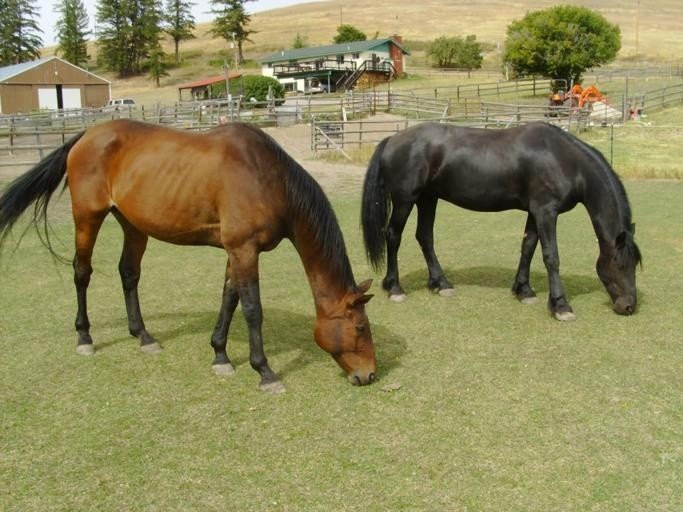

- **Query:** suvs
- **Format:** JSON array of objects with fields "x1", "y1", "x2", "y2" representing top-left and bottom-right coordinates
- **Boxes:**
[{"x1": 100, "y1": 97, "x2": 137, "y2": 110}]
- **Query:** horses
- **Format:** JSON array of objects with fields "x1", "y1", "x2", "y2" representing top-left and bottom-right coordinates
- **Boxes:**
[
  {"x1": 360, "y1": 120, "x2": 644, "y2": 322},
  {"x1": 0, "y1": 119, "x2": 377, "y2": 395}
]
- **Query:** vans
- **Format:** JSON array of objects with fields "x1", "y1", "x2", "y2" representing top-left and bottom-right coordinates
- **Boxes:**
[{"x1": 309, "y1": 78, "x2": 335, "y2": 93}]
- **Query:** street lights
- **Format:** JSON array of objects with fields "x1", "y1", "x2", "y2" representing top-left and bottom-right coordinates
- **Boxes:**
[{"x1": 229, "y1": 32, "x2": 238, "y2": 73}]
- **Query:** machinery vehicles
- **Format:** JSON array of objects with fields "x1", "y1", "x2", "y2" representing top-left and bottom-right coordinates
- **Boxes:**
[{"x1": 546, "y1": 78, "x2": 608, "y2": 116}]
